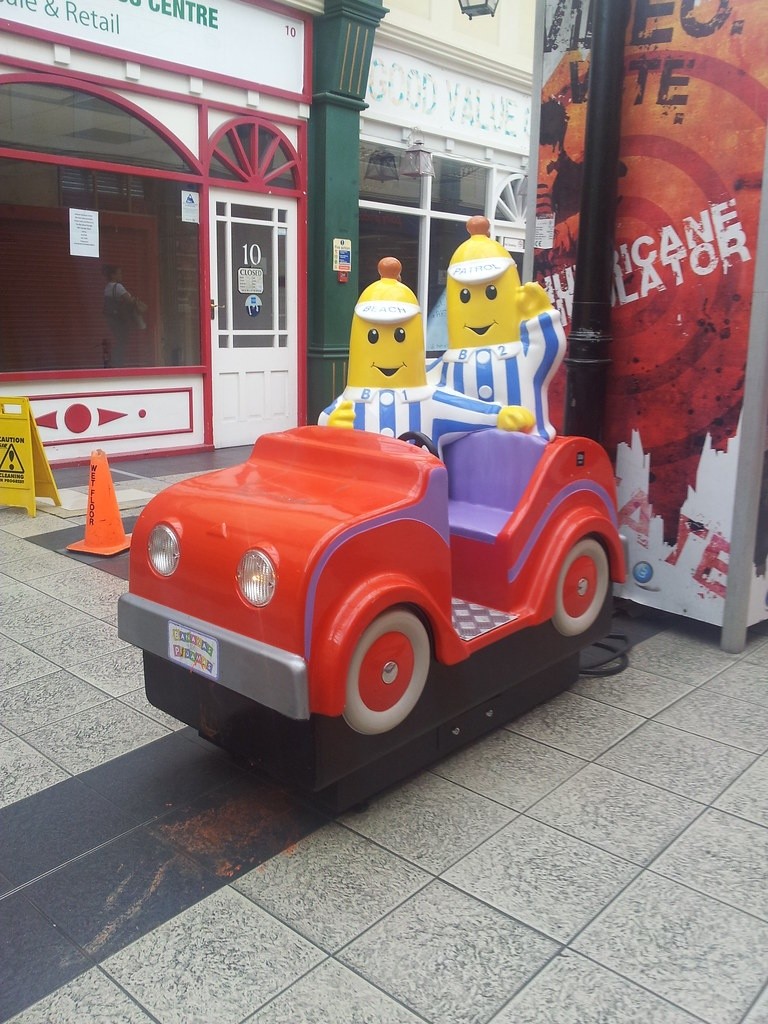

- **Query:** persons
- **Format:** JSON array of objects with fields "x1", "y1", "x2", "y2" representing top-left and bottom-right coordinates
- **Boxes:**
[
  {"x1": 318, "y1": 257, "x2": 538, "y2": 465},
  {"x1": 426, "y1": 215, "x2": 567, "y2": 443},
  {"x1": 101, "y1": 265, "x2": 140, "y2": 367}
]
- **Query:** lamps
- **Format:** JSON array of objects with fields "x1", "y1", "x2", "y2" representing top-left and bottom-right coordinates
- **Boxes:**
[
  {"x1": 365, "y1": 147, "x2": 399, "y2": 182},
  {"x1": 400, "y1": 127, "x2": 435, "y2": 177}
]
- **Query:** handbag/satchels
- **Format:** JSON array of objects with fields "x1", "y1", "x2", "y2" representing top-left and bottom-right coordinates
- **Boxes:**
[{"x1": 102, "y1": 282, "x2": 123, "y2": 320}]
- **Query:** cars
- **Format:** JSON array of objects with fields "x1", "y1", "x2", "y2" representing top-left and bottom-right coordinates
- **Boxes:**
[{"x1": 125, "y1": 389, "x2": 631, "y2": 739}]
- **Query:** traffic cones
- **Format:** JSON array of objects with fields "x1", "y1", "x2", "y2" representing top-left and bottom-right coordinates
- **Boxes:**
[{"x1": 66, "y1": 450, "x2": 133, "y2": 556}]
